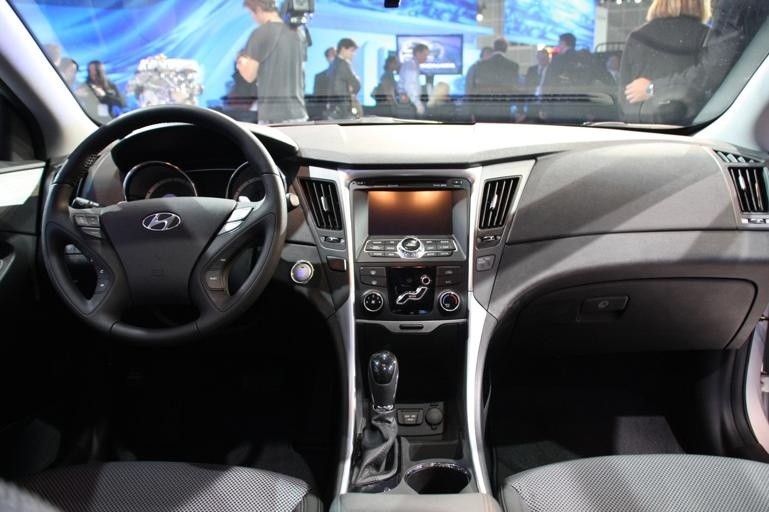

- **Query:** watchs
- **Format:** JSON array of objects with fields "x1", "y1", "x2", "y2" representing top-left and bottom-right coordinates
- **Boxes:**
[{"x1": 646, "y1": 84, "x2": 655, "y2": 97}]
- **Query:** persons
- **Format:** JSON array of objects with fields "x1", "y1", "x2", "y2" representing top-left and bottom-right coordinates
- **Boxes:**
[
  {"x1": 313, "y1": 47, "x2": 336, "y2": 94},
  {"x1": 376, "y1": 44, "x2": 457, "y2": 122},
  {"x1": 327, "y1": 37, "x2": 363, "y2": 119},
  {"x1": 624, "y1": 1, "x2": 767, "y2": 124},
  {"x1": 623, "y1": 1, "x2": 711, "y2": 124},
  {"x1": 237, "y1": 1, "x2": 308, "y2": 127},
  {"x1": 465, "y1": 33, "x2": 621, "y2": 125},
  {"x1": 42, "y1": 45, "x2": 126, "y2": 124}
]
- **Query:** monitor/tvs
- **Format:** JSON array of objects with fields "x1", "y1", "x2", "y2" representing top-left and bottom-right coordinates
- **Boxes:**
[{"x1": 396, "y1": 34, "x2": 462, "y2": 84}]
